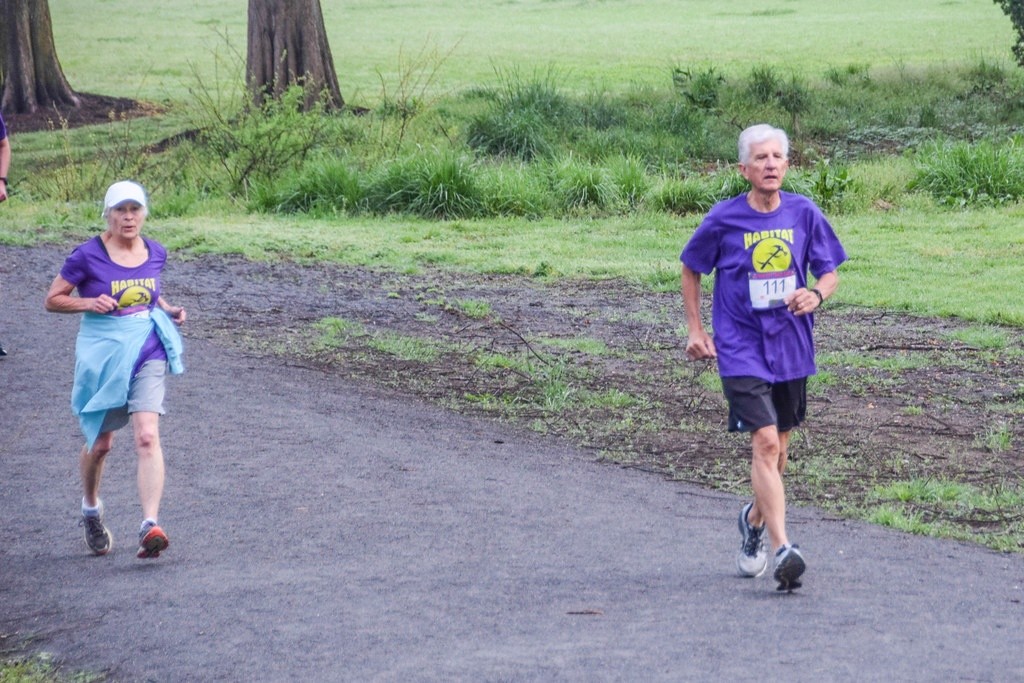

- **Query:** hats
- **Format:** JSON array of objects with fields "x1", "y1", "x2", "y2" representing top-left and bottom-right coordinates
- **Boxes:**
[{"x1": 105, "y1": 180, "x2": 144, "y2": 210}]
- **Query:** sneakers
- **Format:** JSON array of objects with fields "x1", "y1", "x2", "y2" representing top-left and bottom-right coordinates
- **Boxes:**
[
  {"x1": 81, "y1": 498, "x2": 111, "y2": 555},
  {"x1": 138, "y1": 524, "x2": 167, "y2": 559},
  {"x1": 738, "y1": 504, "x2": 769, "y2": 580},
  {"x1": 772, "y1": 542, "x2": 804, "y2": 591}
]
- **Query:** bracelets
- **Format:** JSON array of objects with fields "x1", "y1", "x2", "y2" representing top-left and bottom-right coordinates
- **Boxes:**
[
  {"x1": 0, "y1": 177, "x2": 8, "y2": 184},
  {"x1": 810, "y1": 289, "x2": 824, "y2": 303}
]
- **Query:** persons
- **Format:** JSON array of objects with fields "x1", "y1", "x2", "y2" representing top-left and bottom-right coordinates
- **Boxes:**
[
  {"x1": 46, "y1": 181, "x2": 188, "y2": 557},
  {"x1": 678, "y1": 122, "x2": 845, "y2": 591},
  {"x1": 0, "y1": 114, "x2": 12, "y2": 355}
]
